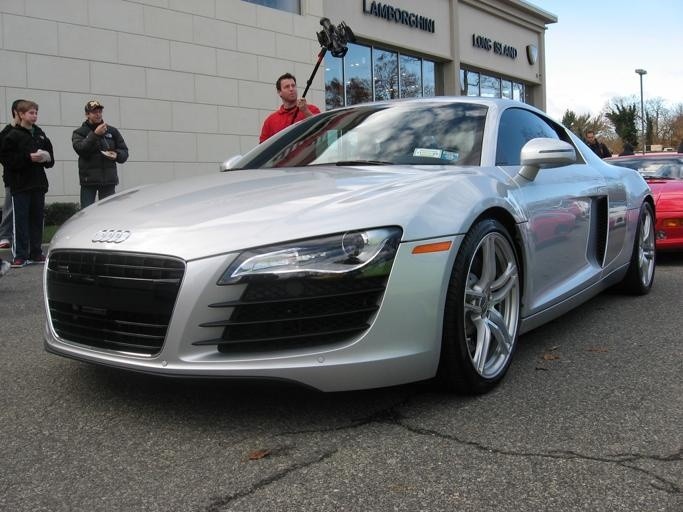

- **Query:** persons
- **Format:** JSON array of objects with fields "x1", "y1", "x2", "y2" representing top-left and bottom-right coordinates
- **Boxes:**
[
  {"x1": 584, "y1": 131, "x2": 612, "y2": 160},
  {"x1": 0, "y1": 99, "x2": 27, "y2": 249},
  {"x1": 72, "y1": 100, "x2": 128, "y2": 211},
  {"x1": 259, "y1": 73, "x2": 321, "y2": 167},
  {"x1": 0, "y1": 101, "x2": 54, "y2": 268}
]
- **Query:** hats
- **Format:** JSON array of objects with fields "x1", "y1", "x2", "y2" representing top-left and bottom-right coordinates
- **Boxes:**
[{"x1": 85, "y1": 100, "x2": 104, "y2": 112}]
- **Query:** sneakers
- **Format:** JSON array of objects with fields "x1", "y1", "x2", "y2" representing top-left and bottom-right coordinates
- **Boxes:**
[
  {"x1": 11, "y1": 258, "x2": 26, "y2": 267},
  {"x1": 0, "y1": 239, "x2": 10, "y2": 248},
  {"x1": 30, "y1": 256, "x2": 46, "y2": 264},
  {"x1": 0, "y1": 261, "x2": 10, "y2": 274}
]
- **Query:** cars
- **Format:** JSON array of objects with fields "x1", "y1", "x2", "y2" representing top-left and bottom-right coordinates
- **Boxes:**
[
  {"x1": 42, "y1": 95, "x2": 658, "y2": 397},
  {"x1": 602, "y1": 153, "x2": 683, "y2": 247}
]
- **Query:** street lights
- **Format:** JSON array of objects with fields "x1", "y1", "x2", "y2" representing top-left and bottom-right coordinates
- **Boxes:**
[{"x1": 636, "y1": 69, "x2": 646, "y2": 153}]
